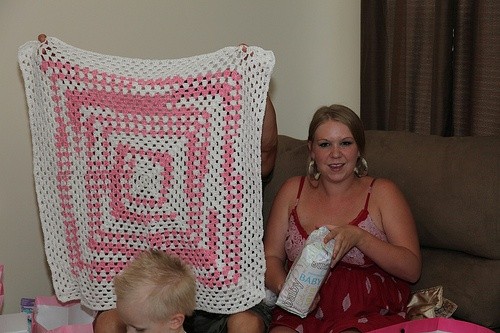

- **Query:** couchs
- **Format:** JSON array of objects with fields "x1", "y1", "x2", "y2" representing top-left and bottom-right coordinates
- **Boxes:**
[{"x1": 262, "y1": 128, "x2": 500, "y2": 328}]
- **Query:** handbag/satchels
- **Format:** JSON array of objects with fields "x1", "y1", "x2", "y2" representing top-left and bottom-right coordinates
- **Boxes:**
[{"x1": 31, "y1": 296, "x2": 100, "y2": 333}]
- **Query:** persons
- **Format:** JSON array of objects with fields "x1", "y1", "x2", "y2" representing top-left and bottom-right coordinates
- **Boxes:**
[
  {"x1": 264, "y1": 104, "x2": 421, "y2": 332},
  {"x1": 116, "y1": 246, "x2": 196, "y2": 333},
  {"x1": 36, "y1": 32, "x2": 278, "y2": 333}
]
